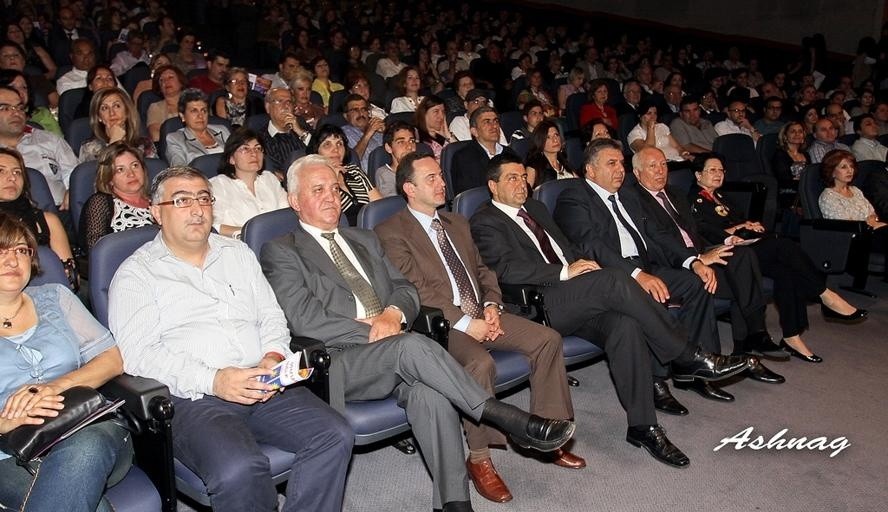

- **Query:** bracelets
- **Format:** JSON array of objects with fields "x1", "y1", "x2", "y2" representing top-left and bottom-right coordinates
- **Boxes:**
[
  {"x1": 299, "y1": 131, "x2": 308, "y2": 141},
  {"x1": 485, "y1": 303, "x2": 504, "y2": 315}
]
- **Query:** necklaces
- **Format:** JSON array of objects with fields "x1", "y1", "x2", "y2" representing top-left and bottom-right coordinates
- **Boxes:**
[{"x1": 0, "y1": 293, "x2": 25, "y2": 330}]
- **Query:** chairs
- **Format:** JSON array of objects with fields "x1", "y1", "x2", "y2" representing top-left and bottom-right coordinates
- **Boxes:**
[
  {"x1": 756, "y1": 133, "x2": 781, "y2": 175},
  {"x1": 109, "y1": 42, "x2": 126, "y2": 57},
  {"x1": 212, "y1": 89, "x2": 267, "y2": 116},
  {"x1": 67, "y1": 117, "x2": 92, "y2": 154},
  {"x1": 499, "y1": 111, "x2": 525, "y2": 142},
  {"x1": 242, "y1": 207, "x2": 450, "y2": 455},
  {"x1": 54, "y1": 64, "x2": 72, "y2": 77},
  {"x1": 162, "y1": 44, "x2": 180, "y2": 52},
  {"x1": 662, "y1": 113, "x2": 678, "y2": 124},
  {"x1": 91, "y1": 226, "x2": 333, "y2": 509},
  {"x1": 28, "y1": 168, "x2": 58, "y2": 211},
  {"x1": 664, "y1": 166, "x2": 774, "y2": 321},
  {"x1": 618, "y1": 114, "x2": 637, "y2": 138},
  {"x1": 28, "y1": 246, "x2": 176, "y2": 512},
  {"x1": 666, "y1": 167, "x2": 695, "y2": 217},
  {"x1": 440, "y1": 139, "x2": 471, "y2": 201},
  {"x1": 454, "y1": 186, "x2": 610, "y2": 387},
  {"x1": 310, "y1": 90, "x2": 323, "y2": 104},
  {"x1": 25, "y1": 66, "x2": 42, "y2": 74},
  {"x1": 533, "y1": 175, "x2": 584, "y2": 217},
  {"x1": 247, "y1": 113, "x2": 306, "y2": 133},
  {"x1": 368, "y1": 143, "x2": 433, "y2": 186},
  {"x1": 799, "y1": 162, "x2": 878, "y2": 302},
  {"x1": 127, "y1": 66, "x2": 148, "y2": 93},
  {"x1": 512, "y1": 139, "x2": 531, "y2": 162},
  {"x1": 711, "y1": 133, "x2": 780, "y2": 223},
  {"x1": 591, "y1": 77, "x2": 621, "y2": 97},
  {"x1": 346, "y1": 146, "x2": 362, "y2": 165},
  {"x1": 160, "y1": 115, "x2": 231, "y2": 160},
  {"x1": 386, "y1": 110, "x2": 418, "y2": 132},
  {"x1": 566, "y1": 92, "x2": 588, "y2": 127},
  {"x1": 358, "y1": 195, "x2": 545, "y2": 394},
  {"x1": 623, "y1": 172, "x2": 638, "y2": 188},
  {"x1": 137, "y1": 90, "x2": 157, "y2": 119},
  {"x1": 188, "y1": 152, "x2": 224, "y2": 179},
  {"x1": 563, "y1": 138, "x2": 584, "y2": 170},
  {"x1": 317, "y1": 114, "x2": 347, "y2": 128},
  {"x1": 57, "y1": 88, "x2": 90, "y2": 128},
  {"x1": 69, "y1": 158, "x2": 168, "y2": 233},
  {"x1": 857, "y1": 161, "x2": 887, "y2": 217},
  {"x1": 330, "y1": 90, "x2": 349, "y2": 114}
]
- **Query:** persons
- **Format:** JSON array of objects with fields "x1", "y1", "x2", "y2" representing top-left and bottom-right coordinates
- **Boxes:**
[
  {"x1": 108, "y1": 166, "x2": 355, "y2": 512},
  {"x1": 79, "y1": 144, "x2": 164, "y2": 257},
  {"x1": 631, "y1": 147, "x2": 791, "y2": 384},
  {"x1": 552, "y1": 137, "x2": 736, "y2": 416},
  {"x1": 317, "y1": 0, "x2": 374, "y2": 113},
  {"x1": 309, "y1": 123, "x2": 384, "y2": 225},
  {"x1": 374, "y1": 151, "x2": 586, "y2": 503},
  {"x1": 468, "y1": 154, "x2": 749, "y2": 468},
  {"x1": 0, "y1": 211, "x2": 134, "y2": 512},
  {"x1": 0, "y1": 147, "x2": 81, "y2": 294},
  {"x1": 342, "y1": 94, "x2": 386, "y2": 168},
  {"x1": 209, "y1": 126, "x2": 291, "y2": 242},
  {"x1": 817, "y1": 148, "x2": 888, "y2": 256},
  {"x1": 259, "y1": 153, "x2": 576, "y2": 512},
  {"x1": 701, "y1": 1, "x2": 888, "y2": 176},
  {"x1": 687, "y1": 151, "x2": 871, "y2": 364}
]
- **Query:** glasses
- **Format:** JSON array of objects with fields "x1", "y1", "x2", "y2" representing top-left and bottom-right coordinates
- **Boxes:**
[
  {"x1": 0, "y1": 103, "x2": 28, "y2": 115},
  {"x1": 235, "y1": 145, "x2": 265, "y2": 154},
  {"x1": 94, "y1": 74, "x2": 114, "y2": 82},
  {"x1": 1, "y1": 248, "x2": 35, "y2": 261},
  {"x1": 471, "y1": 99, "x2": 488, "y2": 105},
  {"x1": 270, "y1": 98, "x2": 293, "y2": 105},
  {"x1": 159, "y1": 195, "x2": 217, "y2": 206},
  {"x1": 729, "y1": 108, "x2": 746, "y2": 113},
  {"x1": 225, "y1": 77, "x2": 250, "y2": 87},
  {"x1": 702, "y1": 167, "x2": 727, "y2": 173}
]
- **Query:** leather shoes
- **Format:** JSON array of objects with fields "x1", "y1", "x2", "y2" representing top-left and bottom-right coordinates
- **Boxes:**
[
  {"x1": 682, "y1": 375, "x2": 736, "y2": 403},
  {"x1": 465, "y1": 455, "x2": 514, "y2": 504},
  {"x1": 548, "y1": 447, "x2": 587, "y2": 469},
  {"x1": 513, "y1": 414, "x2": 576, "y2": 456},
  {"x1": 653, "y1": 381, "x2": 689, "y2": 417},
  {"x1": 819, "y1": 305, "x2": 873, "y2": 325},
  {"x1": 673, "y1": 345, "x2": 750, "y2": 386},
  {"x1": 780, "y1": 337, "x2": 825, "y2": 364},
  {"x1": 747, "y1": 334, "x2": 789, "y2": 360},
  {"x1": 735, "y1": 356, "x2": 786, "y2": 385},
  {"x1": 626, "y1": 425, "x2": 689, "y2": 468}
]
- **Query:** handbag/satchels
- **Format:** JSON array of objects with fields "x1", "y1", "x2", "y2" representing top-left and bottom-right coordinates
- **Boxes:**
[{"x1": 3, "y1": 385, "x2": 106, "y2": 475}]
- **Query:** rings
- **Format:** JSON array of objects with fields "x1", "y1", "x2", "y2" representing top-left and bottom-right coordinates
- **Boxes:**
[{"x1": 27, "y1": 387, "x2": 38, "y2": 395}]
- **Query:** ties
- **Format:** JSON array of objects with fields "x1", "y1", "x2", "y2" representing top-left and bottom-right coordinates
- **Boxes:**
[
  {"x1": 321, "y1": 231, "x2": 382, "y2": 319},
  {"x1": 608, "y1": 194, "x2": 653, "y2": 273},
  {"x1": 431, "y1": 219, "x2": 483, "y2": 318},
  {"x1": 517, "y1": 207, "x2": 564, "y2": 266},
  {"x1": 656, "y1": 191, "x2": 704, "y2": 253}
]
